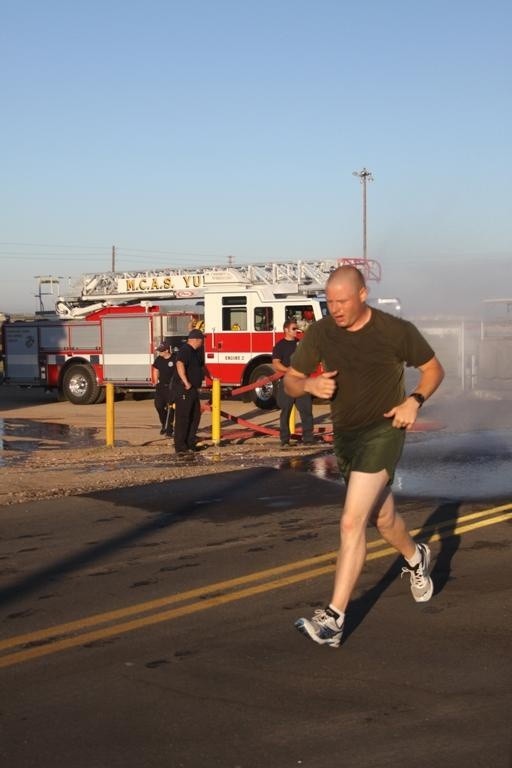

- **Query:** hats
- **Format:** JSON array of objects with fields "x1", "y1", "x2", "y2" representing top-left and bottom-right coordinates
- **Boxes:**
[
  {"x1": 188, "y1": 329, "x2": 206, "y2": 338},
  {"x1": 155, "y1": 342, "x2": 170, "y2": 351}
]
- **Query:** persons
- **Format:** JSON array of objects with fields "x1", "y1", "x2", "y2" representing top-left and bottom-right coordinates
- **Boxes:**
[
  {"x1": 271, "y1": 319, "x2": 319, "y2": 448},
  {"x1": 173, "y1": 328, "x2": 213, "y2": 460},
  {"x1": 281, "y1": 267, "x2": 447, "y2": 651},
  {"x1": 152, "y1": 342, "x2": 176, "y2": 437}
]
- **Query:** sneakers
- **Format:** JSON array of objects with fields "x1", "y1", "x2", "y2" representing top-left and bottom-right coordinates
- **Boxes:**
[
  {"x1": 295, "y1": 605, "x2": 345, "y2": 648},
  {"x1": 400, "y1": 543, "x2": 434, "y2": 602}
]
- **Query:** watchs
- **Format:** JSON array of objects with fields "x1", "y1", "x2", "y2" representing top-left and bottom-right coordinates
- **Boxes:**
[{"x1": 408, "y1": 392, "x2": 425, "y2": 409}]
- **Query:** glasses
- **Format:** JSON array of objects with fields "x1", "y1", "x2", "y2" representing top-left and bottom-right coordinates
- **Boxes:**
[{"x1": 292, "y1": 328, "x2": 297, "y2": 330}]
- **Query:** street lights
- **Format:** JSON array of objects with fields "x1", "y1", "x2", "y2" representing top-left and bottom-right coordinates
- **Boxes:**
[{"x1": 353, "y1": 167, "x2": 375, "y2": 258}]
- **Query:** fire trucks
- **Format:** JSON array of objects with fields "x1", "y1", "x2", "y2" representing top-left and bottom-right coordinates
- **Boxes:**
[{"x1": 0, "y1": 258, "x2": 402, "y2": 409}]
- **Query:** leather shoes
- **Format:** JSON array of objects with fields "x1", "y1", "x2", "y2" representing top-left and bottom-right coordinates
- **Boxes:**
[
  {"x1": 192, "y1": 443, "x2": 207, "y2": 452},
  {"x1": 159, "y1": 426, "x2": 166, "y2": 435}
]
